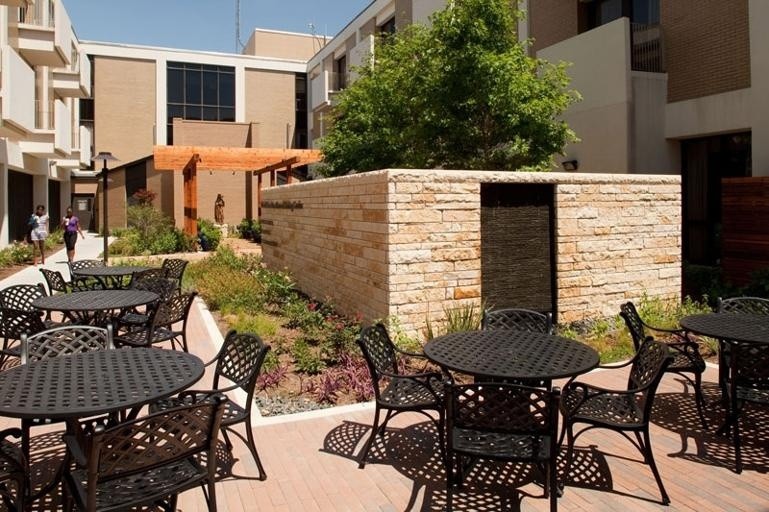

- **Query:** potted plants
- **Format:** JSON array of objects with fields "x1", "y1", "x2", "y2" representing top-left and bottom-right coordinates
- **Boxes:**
[{"x1": 197, "y1": 218, "x2": 223, "y2": 251}]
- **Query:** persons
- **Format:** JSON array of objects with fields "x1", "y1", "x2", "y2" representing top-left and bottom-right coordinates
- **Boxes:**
[
  {"x1": 27, "y1": 205, "x2": 49, "y2": 268},
  {"x1": 61, "y1": 207, "x2": 85, "y2": 264}
]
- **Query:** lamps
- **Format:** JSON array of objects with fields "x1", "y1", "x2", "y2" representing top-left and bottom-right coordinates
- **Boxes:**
[{"x1": 562, "y1": 160, "x2": 578, "y2": 171}]
